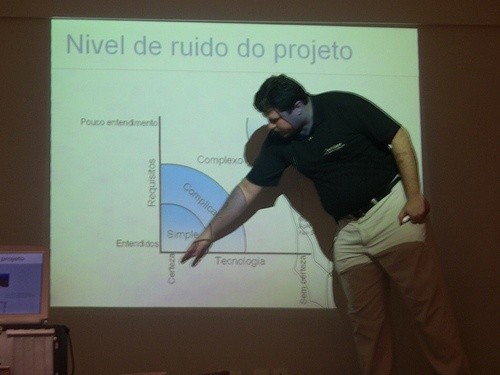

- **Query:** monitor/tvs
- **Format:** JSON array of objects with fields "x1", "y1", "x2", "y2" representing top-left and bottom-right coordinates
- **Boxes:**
[{"x1": 0, "y1": 244, "x2": 50, "y2": 327}]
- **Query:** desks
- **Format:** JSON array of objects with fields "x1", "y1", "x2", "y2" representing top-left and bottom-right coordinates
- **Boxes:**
[{"x1": 0, "y1": 328, "x2": 68, "y2": 375}]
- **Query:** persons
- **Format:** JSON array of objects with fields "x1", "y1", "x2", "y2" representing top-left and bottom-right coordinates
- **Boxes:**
[{"x1": 180, "y1": 74, "x2": 473, "y2": 375}]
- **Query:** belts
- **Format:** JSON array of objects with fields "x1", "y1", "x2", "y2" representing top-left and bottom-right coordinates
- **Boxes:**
[{"x1": 336, "y1": 176, "x2": 402, "y2": 224}]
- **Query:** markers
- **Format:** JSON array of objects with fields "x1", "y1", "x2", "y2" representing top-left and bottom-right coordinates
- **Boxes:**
[{"x1": 400, "y1": 215, "x2": 411, "y2": 224}]
